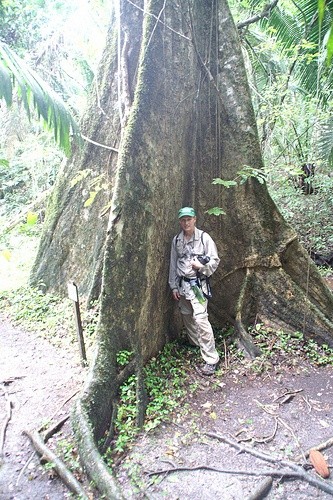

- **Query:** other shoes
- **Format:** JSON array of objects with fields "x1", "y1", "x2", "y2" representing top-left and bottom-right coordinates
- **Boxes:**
[{"x1": 202, "y1": 364, "x2": 217, "y2": 375}]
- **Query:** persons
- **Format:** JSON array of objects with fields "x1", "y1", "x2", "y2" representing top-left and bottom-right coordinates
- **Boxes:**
[{"x1": 167, "y1": 206, "x2": 220, "y2": 378}]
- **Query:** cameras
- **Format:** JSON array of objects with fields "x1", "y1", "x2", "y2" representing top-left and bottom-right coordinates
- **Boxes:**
[{"x1": 197, "y1": 255, "x2": 210, "y2": 265}]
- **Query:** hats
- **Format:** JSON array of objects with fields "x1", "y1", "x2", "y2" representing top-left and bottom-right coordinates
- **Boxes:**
[{"x1": 178, "y1": 207, "x2": 196, "y2": 219}]
